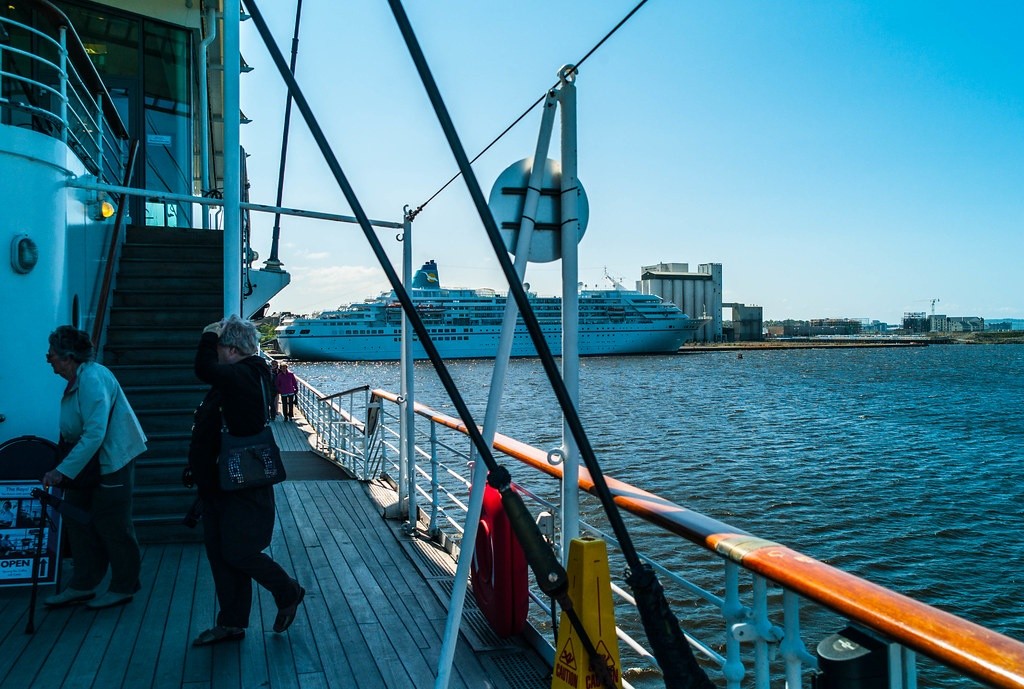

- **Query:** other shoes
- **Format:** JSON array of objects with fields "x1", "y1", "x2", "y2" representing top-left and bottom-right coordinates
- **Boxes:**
[
  {"x1": 86, "y1": 591, "x2": 133, "y2": 610},
  {"x1": 285, "y1": 417, "x2": 288, "y2": 421},
  {"x1": 42, "y1": 586, "x2": 97, "y2": 606},
  {"x1": 272, "y1": 417, "x2": 275, "y2": 420},
  {"x1": 276, "y1": 413, "x2": 279, "y2": 415}
]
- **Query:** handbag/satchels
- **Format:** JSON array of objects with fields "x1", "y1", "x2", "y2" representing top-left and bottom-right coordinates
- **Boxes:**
[
  {"x1": 218, "y1": 373, "x2": 287, "y2": 493},
  {"x1": 57, "y1": 440, "x2": 101, "y2": 487}
]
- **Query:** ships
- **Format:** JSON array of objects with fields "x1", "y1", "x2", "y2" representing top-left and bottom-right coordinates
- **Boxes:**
[{"x1": 274, "y1": 260, "x2": 713, "y2": 361}]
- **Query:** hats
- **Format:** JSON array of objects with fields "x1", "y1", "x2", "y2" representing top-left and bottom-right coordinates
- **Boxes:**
[{"x1": 49, "y1": 325, "x2": 93, "y2": 355}]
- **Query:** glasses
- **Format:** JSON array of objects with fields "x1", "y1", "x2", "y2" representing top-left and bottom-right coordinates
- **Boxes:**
[{"x1": 46, "y1": 353, "x2": 58, "y2": 360}]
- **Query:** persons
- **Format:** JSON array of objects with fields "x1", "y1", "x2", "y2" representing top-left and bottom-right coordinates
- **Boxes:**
[
  {"x1": 41, "y1": 326, "x2": 147, "y2": 609},
  {"x1": 187, "y1": 316, "x2": 306, "y2": 648},
  {"x1": 265, "y1": 360, "x2": 299, "y2": 422}
]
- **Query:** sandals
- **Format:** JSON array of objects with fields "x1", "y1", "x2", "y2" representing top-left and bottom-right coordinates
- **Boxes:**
[
  {"x1": 273, "y1": 587, "x2": 305, "y2": 633},
  {"x1": 193, "y1": 626, "x2": 245, "y2": 647}
]
